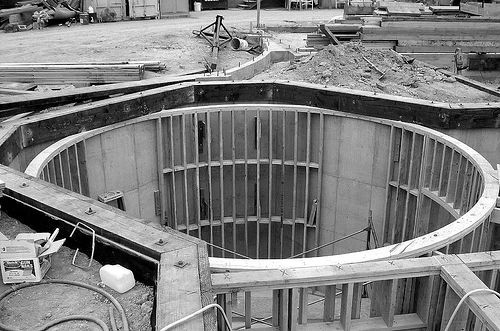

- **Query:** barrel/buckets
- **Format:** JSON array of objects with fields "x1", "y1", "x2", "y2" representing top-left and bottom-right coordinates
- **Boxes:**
[
  {"x1": 230, "y1": 38, "x2": 248, "y2": 51},
  {"x1": 194, "y1": 2, "x2": 202, "y2": 12},
  {"x1": 80, "y1": 14, "x2": 89, "y2": 24},
  {"x1": 88, "y1": 12, "x2": 97, "y2": 24}
]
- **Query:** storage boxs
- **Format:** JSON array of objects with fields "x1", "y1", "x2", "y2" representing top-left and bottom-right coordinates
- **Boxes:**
[{"x1": 1, "y1": 228, "x2": 66, "y2": 286}]
